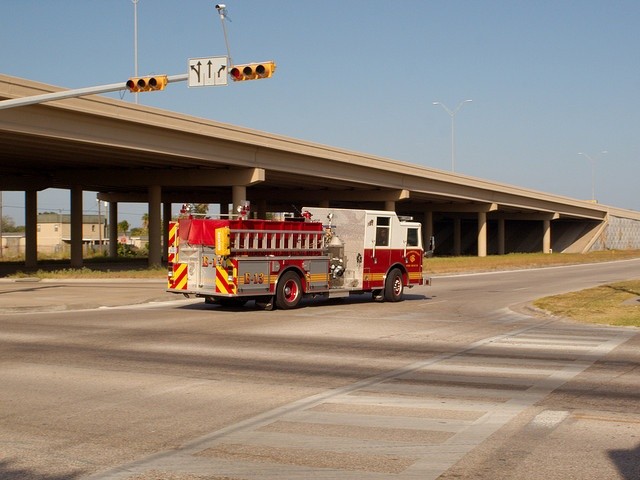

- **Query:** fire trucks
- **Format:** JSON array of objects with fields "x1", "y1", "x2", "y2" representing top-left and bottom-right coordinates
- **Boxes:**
[{"x1": 167, "y1": 201, "x2": 435, "y2": 311}]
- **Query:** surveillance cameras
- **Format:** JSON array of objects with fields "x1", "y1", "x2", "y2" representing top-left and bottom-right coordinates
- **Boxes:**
[{"x1": 215, "y1": 4, "x2": 226, "y2": 10}]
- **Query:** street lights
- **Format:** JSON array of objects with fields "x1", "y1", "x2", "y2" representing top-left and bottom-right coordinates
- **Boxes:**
[
  {"x1": 433, "y1": 99, "x2": 472, "y2": 171},
  {"x1": 578, "y1": 151, "x2": 606, "y2": 199}
]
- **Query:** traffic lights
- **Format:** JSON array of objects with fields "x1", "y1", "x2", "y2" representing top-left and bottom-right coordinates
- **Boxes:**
[
  {"x1": 126, "y1": 74, "x2": 168, "y2": 92},
  {"x1": 230, "y1": 61, "x2": 276, "y2": 81}
]
